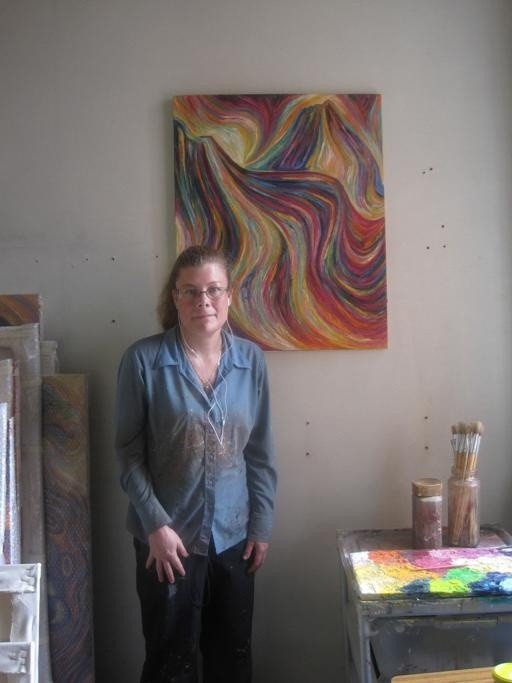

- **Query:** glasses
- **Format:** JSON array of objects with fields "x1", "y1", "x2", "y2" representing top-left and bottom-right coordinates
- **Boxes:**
[{"x1": 173, "y1": 286, "x2": 229, "y2": 300}]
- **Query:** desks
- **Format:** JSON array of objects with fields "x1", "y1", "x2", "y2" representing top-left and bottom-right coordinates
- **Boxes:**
[{"x1": 336, "y1": 523, "x2": 512, "y2": 683}]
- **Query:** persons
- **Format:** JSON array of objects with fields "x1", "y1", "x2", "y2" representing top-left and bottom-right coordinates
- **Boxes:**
[{"x1": 111, "y1": 244, "x2": 280, "y2": 683}]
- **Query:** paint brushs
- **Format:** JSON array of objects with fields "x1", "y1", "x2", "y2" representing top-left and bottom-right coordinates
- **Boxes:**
[{"x1": 449, "y1": 421, "x2": 485, "y2": 547}]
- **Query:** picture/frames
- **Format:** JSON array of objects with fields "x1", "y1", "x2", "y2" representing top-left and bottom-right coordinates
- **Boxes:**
[{"x1": 172, "y1": 93, "x2": 390, "y2": 354}]
